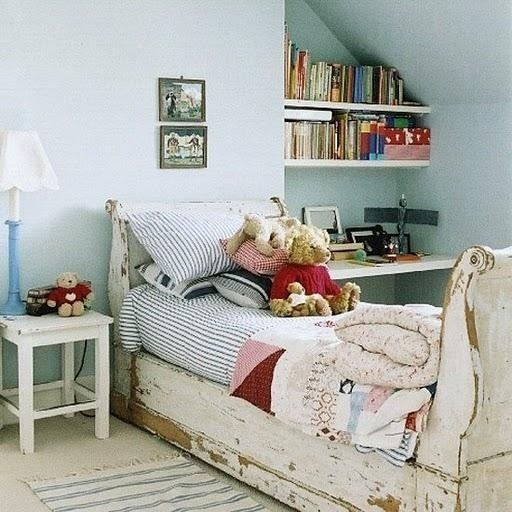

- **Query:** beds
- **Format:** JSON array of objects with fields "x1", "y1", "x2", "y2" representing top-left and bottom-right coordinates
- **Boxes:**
[{"x1": 105, "y1": 190, "x2": 510, "y2": 511}]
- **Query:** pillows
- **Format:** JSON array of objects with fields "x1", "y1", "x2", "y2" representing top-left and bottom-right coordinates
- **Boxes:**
[
  {"x1": 132, "y1": 258, "x2": 222, "y2": 302},
  {"x1": 216, "y1": 233, "x2": 292, "y2": 280},
  {"x1": 207, "y1": 266, "x2": 279, "y2": 309},
  {"x1": 117, "y1": 201, "x2": 256, "y2": 289}
]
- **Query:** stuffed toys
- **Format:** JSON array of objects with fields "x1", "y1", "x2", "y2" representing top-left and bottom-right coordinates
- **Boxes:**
[
  {"x1": 269, "y1": 228, "x2": 362, "y2": 318},
  {"x1": 287, "y1": 281, "x2": 309, "y2": 317},
  {"x1": 47, "y1": 272, "x2": 94, "y2": 317},
  {"x1": 225, "y1": 211, "x2": 301, "y2": 257}
]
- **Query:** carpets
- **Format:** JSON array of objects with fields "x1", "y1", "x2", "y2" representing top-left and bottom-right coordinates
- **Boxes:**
[{"x1": 16, "y1": 448, "x2": 274, "y2": 511}]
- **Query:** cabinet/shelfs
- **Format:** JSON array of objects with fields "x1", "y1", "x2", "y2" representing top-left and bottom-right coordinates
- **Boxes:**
[{"x1": 282, "y1": 89, "x2": 434, "y2": 169}]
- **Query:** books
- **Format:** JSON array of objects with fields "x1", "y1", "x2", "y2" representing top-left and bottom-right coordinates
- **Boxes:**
[
  {"x1": 327, "y1": 242, "x2": 365, "y2": 250},
  {"x1": 331, "y1": 251, "x2": 357, "y2": 261},
  {"x1": 284, "y1": 20, "x2": 417, "y2": 159}
]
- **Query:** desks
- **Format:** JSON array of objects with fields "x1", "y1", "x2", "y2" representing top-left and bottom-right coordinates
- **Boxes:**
[
  {"x1": 321, "y1": 247, "x2": 463, "y2": 283},
  {"x1": 0, "y1": 307, "x2": 119, "y2": 457}
]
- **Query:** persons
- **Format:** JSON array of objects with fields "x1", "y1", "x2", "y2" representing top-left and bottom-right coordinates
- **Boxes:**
[{"x1": 384, "y1": 241, "x2": 399, "y2": 264}]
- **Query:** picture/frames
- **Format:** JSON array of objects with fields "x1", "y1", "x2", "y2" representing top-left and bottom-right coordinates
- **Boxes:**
[
  {"x1": 158, "y1": 75, "x2": 207, "y2": 124},
  {"x1": 301, "y1": 203, "x2": 413, "y2": 257},
  {"x1": 159, "y1": 124, "x2": 209, "y2": 170}
]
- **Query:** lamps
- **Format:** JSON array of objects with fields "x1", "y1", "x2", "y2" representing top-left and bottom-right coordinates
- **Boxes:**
[{"x1": 1, "y1": 124, "x2": 66, "y2": 317}]
